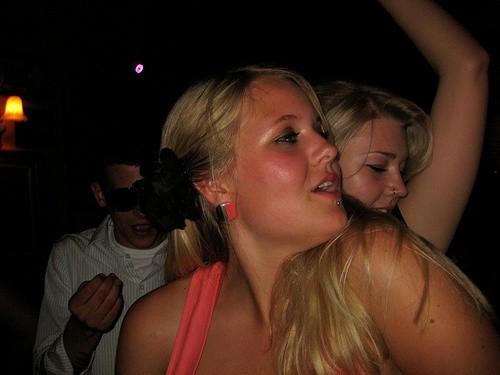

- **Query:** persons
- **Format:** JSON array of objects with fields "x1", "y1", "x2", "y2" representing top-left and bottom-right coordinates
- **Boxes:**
[
  {"x1": 114, "y1": 69, "x2": 500, "y2": 375},
  {"x1": 312, "y1": 0, "x2": 489, "y2": 255},
  {"x1": 33, "y1": 144, "x2": 170, "y2": 375}
]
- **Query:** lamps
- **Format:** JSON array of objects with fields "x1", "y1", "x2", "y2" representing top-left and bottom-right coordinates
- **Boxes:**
[{"x1": 1, "y1": 80, "x2": 28, "y2": 122}]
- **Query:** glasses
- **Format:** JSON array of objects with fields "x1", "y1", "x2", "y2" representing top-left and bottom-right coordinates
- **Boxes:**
[{"x1": 98, "y1": 182, "x2": 139, "y2": 213}]
